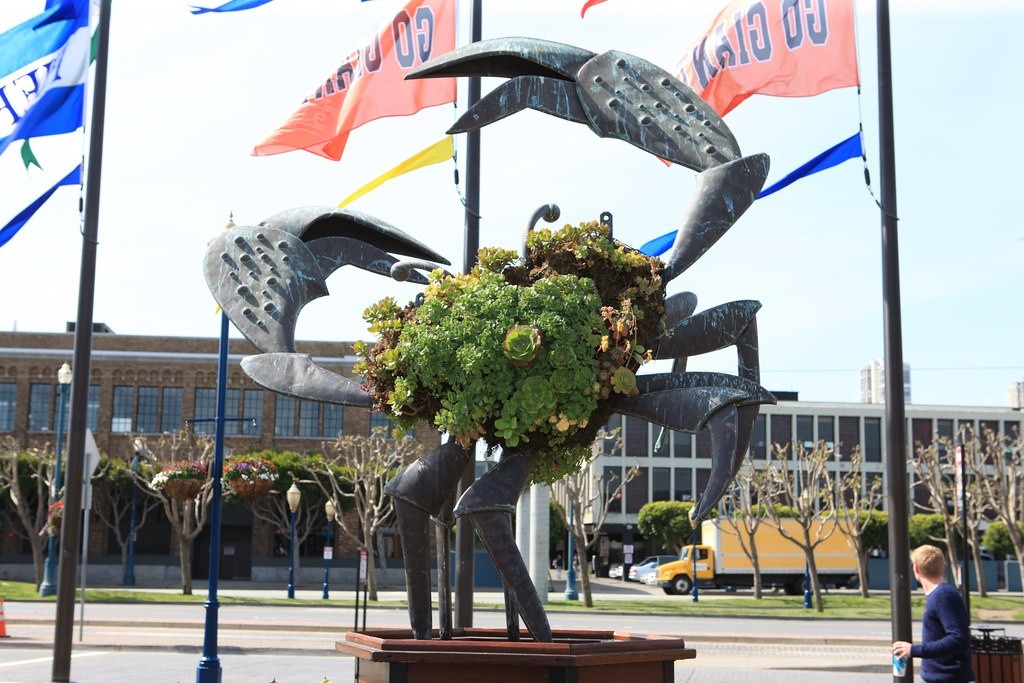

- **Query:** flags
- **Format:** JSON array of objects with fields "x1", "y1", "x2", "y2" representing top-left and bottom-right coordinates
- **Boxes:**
[
  {"x1": 0, "y1": 0, "x2": 92, "y2": 152},
  {"x1": 658, "y1": 0, "x2": 860, "y2": 167},
  {"x1": 251, "y1": 0, "x2": 457, "y2": 161}
]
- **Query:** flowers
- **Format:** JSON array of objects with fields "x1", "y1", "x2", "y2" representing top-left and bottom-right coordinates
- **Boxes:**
[
  {"x1": 223, "y1": 457, "x2": 280, "y2": 485},
  {"x1": 49, "y1": 501, "x2": 63, "y2": 519},
  {"x1": 148, "y1": 461, "x2": 206, "y2": 491}
]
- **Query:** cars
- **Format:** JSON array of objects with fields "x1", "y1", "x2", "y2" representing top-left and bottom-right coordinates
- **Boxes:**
[{"x1": 627, "y1": 556, "x2": 657, "y2": 585}]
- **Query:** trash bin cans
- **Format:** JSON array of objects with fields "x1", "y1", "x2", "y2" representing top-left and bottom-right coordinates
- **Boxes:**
[{"x1": 969, "y1": 626, "x2": 1024, "y2": 683}]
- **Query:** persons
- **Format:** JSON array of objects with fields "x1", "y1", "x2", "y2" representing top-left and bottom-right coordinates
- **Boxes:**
[
  {"x1": 892, "y1": 545, "x2": 976, "y2": 683},
  {"x1": 556, "y1": 555, "x2": 562, "y2": 578},
  {"x1": 697, "y1": 550, "x2": 707, "y2": 559}
]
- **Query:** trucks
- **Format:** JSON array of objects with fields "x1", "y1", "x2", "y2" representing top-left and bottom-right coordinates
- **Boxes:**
[{"x1": 654, "y1": 516, "x2": 860, "y2": 596}]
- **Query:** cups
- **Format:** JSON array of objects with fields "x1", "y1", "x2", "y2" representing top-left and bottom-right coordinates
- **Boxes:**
[{"x1": 892, "y1": 651, "x2": 907, "y2": 677}]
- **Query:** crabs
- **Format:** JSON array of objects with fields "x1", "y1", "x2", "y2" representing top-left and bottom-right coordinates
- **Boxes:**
[{"x1": 201, "y1": 35, "x2": 778, "y2": 643}]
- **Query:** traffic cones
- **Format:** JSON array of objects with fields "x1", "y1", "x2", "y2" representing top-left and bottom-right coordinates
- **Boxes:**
[{"x1": 0, "y1": 598, "x2": 11, "y2": 639}]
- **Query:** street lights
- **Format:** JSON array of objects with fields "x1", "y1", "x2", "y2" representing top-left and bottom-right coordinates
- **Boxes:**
[
  {"x1": 687, "y1": 504, "x2": 699, "y2": 603},
  {"x1": 286, "y1": 482, "x2": 302, "y2": 597},
  {"x1": 39, "y1": 363, "x2": 74, "y2": 598},
  {"x1": 122, "y1": 436, "x2": 143, "y2": 586},
  {"x1": 322, "y1": 496, "x2": 337, "y2": 598}
]
